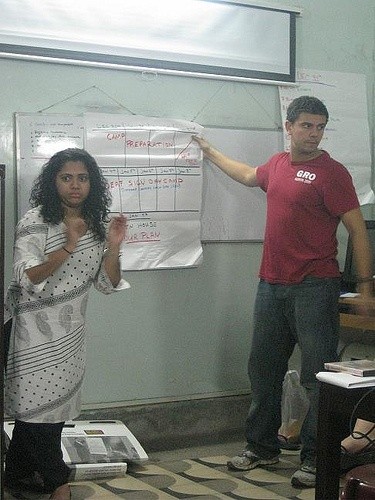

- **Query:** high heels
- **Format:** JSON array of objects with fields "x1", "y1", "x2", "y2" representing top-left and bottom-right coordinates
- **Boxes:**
[{"x1": 339, "y1": 431, "x2": 375, "y2": 468}]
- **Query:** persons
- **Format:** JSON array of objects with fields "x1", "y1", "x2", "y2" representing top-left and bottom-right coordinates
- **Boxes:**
[
  {"x1": 73, "y1": 437, "x2": 90, "y2": 463},
  {"x1": 131, "y1": 447, "x2": 140, "y2": 463},
  {"x1": 190, "y1": 95, "x2": 375, "y2": 489},
  {"x1": 5, "y1": 148, "x2": 131, "y2": 500}
]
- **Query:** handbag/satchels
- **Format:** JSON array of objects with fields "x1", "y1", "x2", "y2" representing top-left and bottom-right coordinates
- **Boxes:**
[{"x1": 279, "y1": 370, "x2": 309, "y2": 442}]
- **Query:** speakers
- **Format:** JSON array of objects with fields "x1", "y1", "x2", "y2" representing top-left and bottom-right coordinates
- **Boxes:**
[{"x1": 343, "y1": 220, "x2": 375, "y2": 293}]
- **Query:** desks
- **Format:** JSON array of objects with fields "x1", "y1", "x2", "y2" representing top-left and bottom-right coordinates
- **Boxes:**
[
  {"x1": 338, "y1": 295, "x2": 375, "y2": 331},
  {"x1": 314, "y1": 373, "x2": 375, "y2": 500}
]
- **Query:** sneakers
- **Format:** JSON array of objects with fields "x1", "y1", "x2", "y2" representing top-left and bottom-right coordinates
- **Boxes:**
[
  {"x1": 227, "y1": 449, "x2": 279, "y2": 470},
  {"x1": 291, "y1": 464, "x2": 316, "y2": 487}
]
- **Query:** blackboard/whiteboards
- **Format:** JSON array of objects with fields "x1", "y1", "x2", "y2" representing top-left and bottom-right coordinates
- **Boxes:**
[
  {"x1": 16, "y1": 112, "x2": 160, "y2": 223},
  {"x1": 201, "y1": 125, "x2": 284, "y2": 243}
]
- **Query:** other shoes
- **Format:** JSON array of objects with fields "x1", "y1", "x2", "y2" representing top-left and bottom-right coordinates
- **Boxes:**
[
  {"x1": 50, "y1": 484, "x2": 71, "y2": 500},
  {"x1": 11, "y1": 472, "x2": 45, "y2": 490}
]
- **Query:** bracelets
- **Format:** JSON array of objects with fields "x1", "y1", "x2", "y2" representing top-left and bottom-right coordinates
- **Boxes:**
[
  {"x1": 358, "y1": 275, "x2": 372, "y2": 283},
  {"x1": 62, "y1": 246, "x2": 73, "y2": 255}
]
